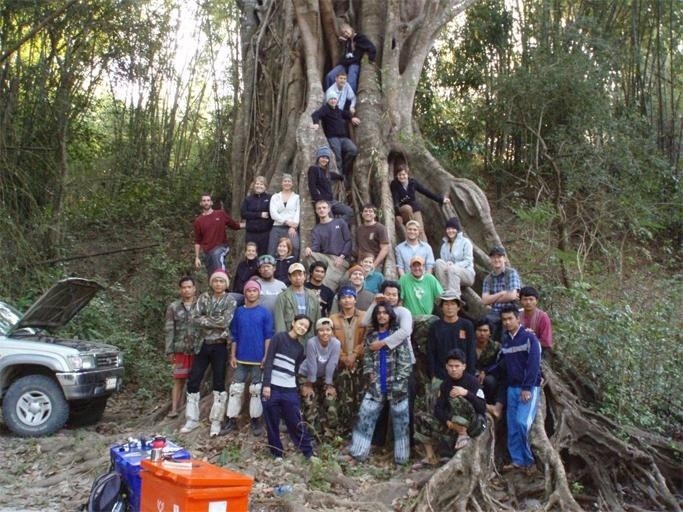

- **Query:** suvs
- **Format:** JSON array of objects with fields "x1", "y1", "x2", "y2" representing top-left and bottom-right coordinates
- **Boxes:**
[{"x1": 0, "y1": 272, "x2": 125, "y2": 436}]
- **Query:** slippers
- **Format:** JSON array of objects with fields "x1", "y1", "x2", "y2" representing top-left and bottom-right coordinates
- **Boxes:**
[
  {"x1": 527, "y1": 464, "x2": 537, "y2": 476},
  {"x1": 485, "y1": 402, "x2": 500, "y2": 419},
  {"x1": 503, "y1": 463, "x2": 521, "y2": 469},
  {"x1": 167, "y1": 410, "x2": 178, "y2": 418},
  {"x1": 454, "y1": 435, "x2": 471, "y2": 450},
  {"x1": 411, "y1": 459, "x2": 432, "y2": 469}
]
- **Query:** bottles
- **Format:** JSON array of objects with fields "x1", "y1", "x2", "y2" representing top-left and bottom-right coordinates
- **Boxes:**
[{"x1": 139, "y1": 433, "x2": 145, "y2": 447}]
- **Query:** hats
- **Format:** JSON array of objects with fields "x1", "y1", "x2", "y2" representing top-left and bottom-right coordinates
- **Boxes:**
[
  {"x1": 327, "y1": 92, "x2": 337, "y2": 101},
  {"x1": 317, "y1": 147, "x2": 331, "y2": 159},
  {"x1": 488, "y1": 246, "x2": 505, "y2": 256},
  {"x1": 257, "y1": 255, "x2": 275, "y2": 268},
  {"x1": 288, "y1": 263, "x2": 305, "y2": 273},
  {"x1": 348, "y1": 265, "x2": 364, "y2": 278},
  {"x1": 410, "y1": 256, "x2": 424, "y2": 266},
  {"x1": 243, "y1": 281, "x2": 260, "y2": 295},
  {"x1": 209, "y1": 269, "x2": 229, "y2": 289},
  {"x1": 446, "y1": 217, "x2": 460, "y2": 231},
  {"x1": 316, "y1": 317, "x2": 333, "y2": 330},
  {"x1": 337, "y1": 285, "x2": 357, "y2": 299},
  {"x1": 435, "y1": 290, "x2": 465, "y2": 305}
]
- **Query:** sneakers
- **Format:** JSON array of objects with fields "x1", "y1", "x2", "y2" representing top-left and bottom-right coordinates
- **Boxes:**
[
  {"x1": 208, "y1": 420, "x2": 220, "y2": 437},
  {"x1": 249, "y1": 419, "x2": 263, "y2": 437},
  {"x1": 220, "y1": 419, "x2": 240, "y2": 435},
  {"x1": 181, "y1": 419, "x2": 202, "y2": 432},
  {"x1": 304, "y1": 456, "x2": 323, "y2": 466},
  {"x1": 273, "y1": 455, "x2": 283, "y2": 469}
]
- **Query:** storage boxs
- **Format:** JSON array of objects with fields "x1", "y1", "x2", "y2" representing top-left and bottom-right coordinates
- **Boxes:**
[
  {"x1": 109, "y1": 437, "x2": 190, "y2": 511},
  {"x1": 136, "y1": 455, "x2": 254, "y2": 512}
]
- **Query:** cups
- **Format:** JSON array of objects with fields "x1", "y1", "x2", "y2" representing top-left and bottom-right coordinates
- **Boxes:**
[{"x1": 150, "y1": 449, "x2": 162, "y2": 460}]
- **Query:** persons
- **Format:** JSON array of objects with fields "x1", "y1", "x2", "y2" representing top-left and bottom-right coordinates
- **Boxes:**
[
  {"x1": 312, "y1": 91, "x2": 360, "y2": 177},
  {"x1": 326, "y1": 23, "x2": 376, "y2": 97},
  {"x1": 326, "y1": 72, "x2": 357, "y2": 116}
]
